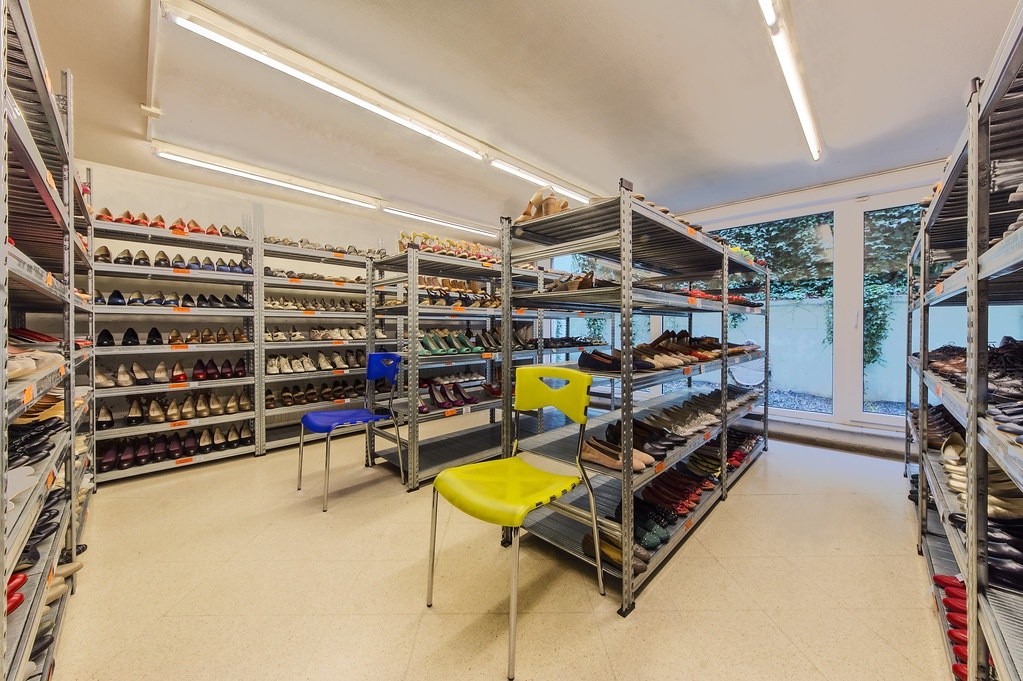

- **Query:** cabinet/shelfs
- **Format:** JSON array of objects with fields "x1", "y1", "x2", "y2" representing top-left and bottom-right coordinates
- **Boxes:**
[{"x1": 0, "y1": 0, "x2": 1023, "y2": 681}]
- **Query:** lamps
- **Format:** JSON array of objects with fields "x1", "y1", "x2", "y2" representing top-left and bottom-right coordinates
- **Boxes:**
[
  {"x1": 147, "y1": 139, "x2": 380, "y2": 212},
  {"x1": 758, "y1": 0, "x2": 791, "y2": 29},
  {"x1": 487, "y1": 145, "x2": 601, "y2": 203},
  {"x1": 766, "y1": 23, "x2": 822, "y2": 159},
  {"x1": 159, "y1": 1, "x2": 488, "y2": 161},
  {"x1": 379, "y1": 201, "x2": 502, "y2": 240}
]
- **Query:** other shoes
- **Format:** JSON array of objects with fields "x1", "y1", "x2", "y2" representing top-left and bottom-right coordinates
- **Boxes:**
[
  {"x1": 87, "y1": 358, "x2": 247, "y2": 388},
  {"x1": 99, "y1": 422, "x2": 254, "y2": 473},
  {"x1": 95, "y1": 206, "x2": 252, "y2": 240},
  {"x1": 95, "y1": 327, "x2": 250, "y2": 346},
  {"x1": 509, "y1": 184, "x2": 768, "y2": 372},
  {"x1": 913, "y1": 336, "x2": 1023, "y2": 447},
  {"x1": 910, "y1": 151, "x2": 1023, "y2": 300},
  {"x1": 940, "y1": 432, "x2": 1023, "y2": 595},
  {"x1": 932, "y1": 572, "x2": 999, "y2": 681},
  {"x1": 6, "y1": 385, "x2": 93, "y2": 513},
  {"x1": 94, "y1": 245, "x2": 254, "y2": 276},
  {"x1": 582, "y1": 383, "x2": 765, "y2": 573},
  {"x1": 5, "y1": 431, "x2": 95, "y2": 681},
  {"x1": 909, "y1": 403, "x2": 966, "y2": 450},
  {"x1": 265, "y1": 229, "x2": 612, "y2": 412},
  {"x1": 909, "y1": 472, "x2": 937, "y2": 511},
  {"x1": 95, "y1": 390, "x2": 253, "y2": 430},
  {"x1": 95, "y1": 287, "x2": 254, "y2": 310},
  {"x1": 7, "y1": 326, "x2": 65, "y2": 380}
]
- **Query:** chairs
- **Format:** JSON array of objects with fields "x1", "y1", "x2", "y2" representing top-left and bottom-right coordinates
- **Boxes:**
[
  {"x1": 426, "y1": 363, "x2": 606, "y2": 681},
  {"x1": 297, "y1": 353, "x2": 405, "y2": 512}
]
